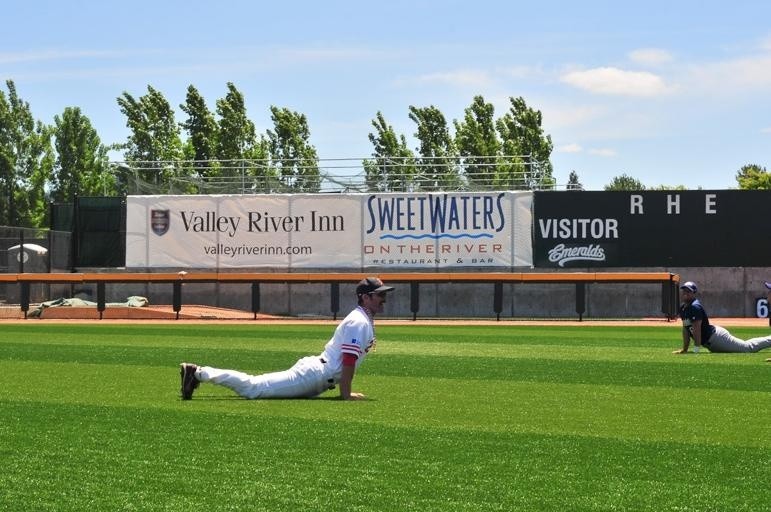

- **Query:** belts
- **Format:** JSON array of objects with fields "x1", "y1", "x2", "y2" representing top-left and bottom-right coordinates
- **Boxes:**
[{"x1": 320, "y1": 359, "x2": 335, "y2": 389}]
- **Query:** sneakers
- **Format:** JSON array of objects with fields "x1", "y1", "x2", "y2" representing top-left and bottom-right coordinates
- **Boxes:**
[{"x1": 180, "y1": 363, "x2": 199, "y2": 401}]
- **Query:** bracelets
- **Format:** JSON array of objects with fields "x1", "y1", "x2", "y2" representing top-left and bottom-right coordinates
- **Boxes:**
[{"x1": 693, "y1": 346, "x2": 700, "y2": 353}]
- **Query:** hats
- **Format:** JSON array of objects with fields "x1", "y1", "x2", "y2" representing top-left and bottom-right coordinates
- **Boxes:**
[
  {"x1": 355, "y1": 277, "x2": 394, "y2": 293},
  {"x1": 680, "y1": 281, "x2": 697, "y2": 294}
]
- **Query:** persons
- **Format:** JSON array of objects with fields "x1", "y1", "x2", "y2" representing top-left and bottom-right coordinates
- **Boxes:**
[
  {"x1": 178, "y1": 276, "x2": 397, "y2": 403},
  {"x1": 672, "y1": 281, "x2": 771, "y2": 356}
]
